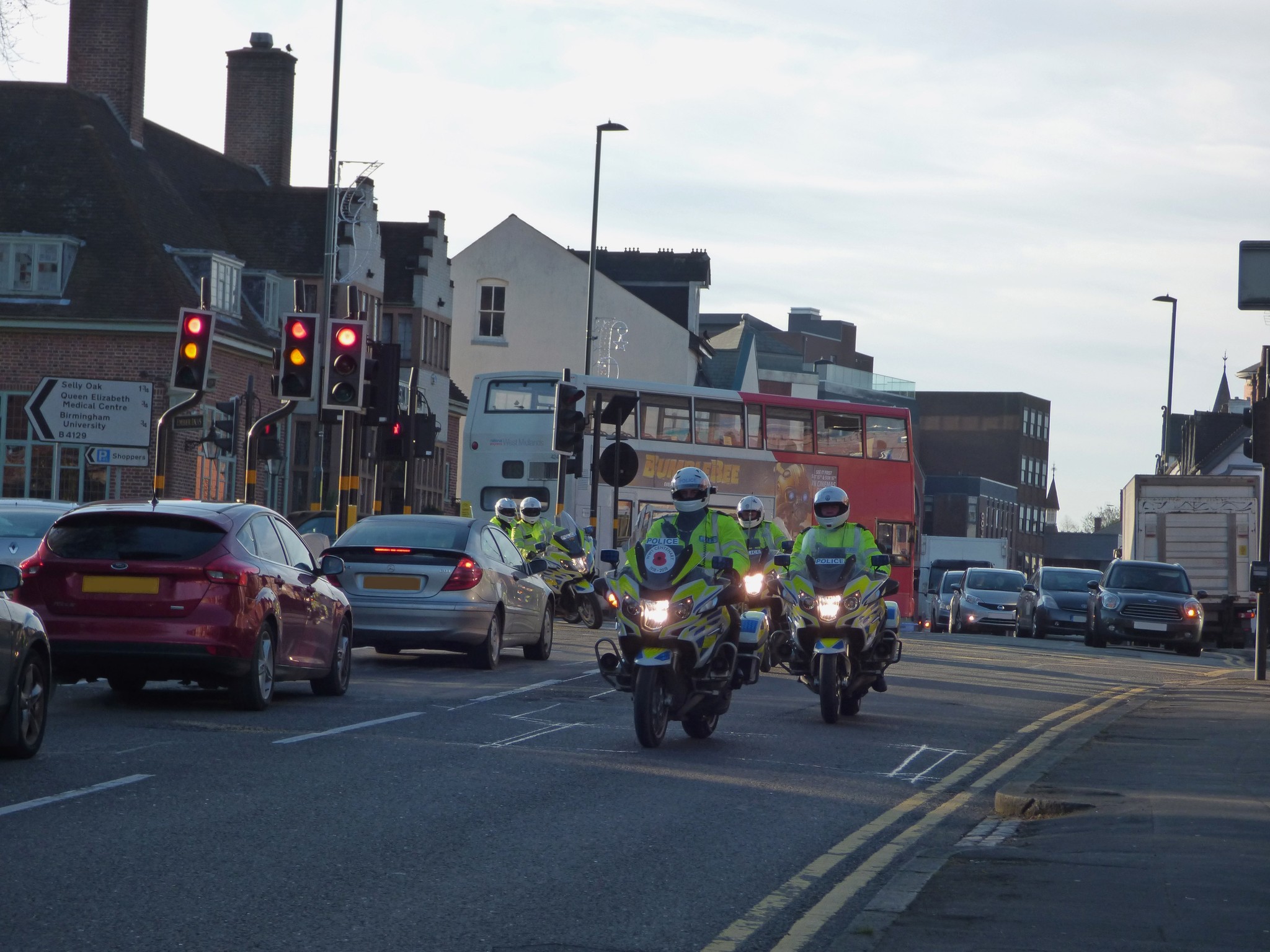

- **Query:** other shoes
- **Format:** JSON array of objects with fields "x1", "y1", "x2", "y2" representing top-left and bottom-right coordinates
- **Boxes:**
[{"x1": 871, "y1": 674, "x2": 888, "y2": 693}]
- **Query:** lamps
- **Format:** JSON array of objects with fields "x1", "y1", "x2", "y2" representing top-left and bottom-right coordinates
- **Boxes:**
[
  {"x1": 435, "y1": 420, "x2": 442, "y2": 444},
  {"x1": 185, "y1": 420, "x2": 221, "y2": 458},
  {"x1": 257, "y1": 438, "x2": 287, "y2": 476}
]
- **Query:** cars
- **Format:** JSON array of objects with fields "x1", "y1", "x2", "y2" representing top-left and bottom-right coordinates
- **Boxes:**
[
  {"x1": 0, "y1": 494, "x2": 557, "y2": 758},
  {"x1": 935, "y1": 557, "x2": 1206, "y2": 655}
]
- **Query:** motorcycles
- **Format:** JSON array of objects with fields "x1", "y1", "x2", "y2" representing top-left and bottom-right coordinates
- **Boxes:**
[{"x1": 523, "y1": 529, "x2": 906, "y2": 747}]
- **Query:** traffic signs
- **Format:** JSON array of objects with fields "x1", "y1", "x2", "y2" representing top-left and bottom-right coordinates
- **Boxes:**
[{"x1": 24, "y1": 376, "x2": 154, "y2": 470}]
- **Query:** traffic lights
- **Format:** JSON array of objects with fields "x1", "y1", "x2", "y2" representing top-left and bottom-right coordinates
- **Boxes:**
[
  {"x1": 1241, "y1": 401, "x2": 1269, "y2": 462},
  {"x1": 393, "y1": 421, "x2": 407, "y2": 438},
  {"x1": 258, "y1": 416, "x2": 279, "y2": 461},
  {"x1": 214, "y1": 396, "x2": 238, "y2": 455},
  {"x1": 170, "y1": 307, "x2": 217, "y2": 395},
  {"x1": 364, "y1": 355, "x2": 381, "y2": 417},
  {"x1": 322, "y1": 317, "x2": 367, "y2": 415},
  {"x1": 272, "y1": 312, "x2": 321, "y2": 399},
  {"x1": 549, "y1": 381, "x2": 586, "y2": 457}
]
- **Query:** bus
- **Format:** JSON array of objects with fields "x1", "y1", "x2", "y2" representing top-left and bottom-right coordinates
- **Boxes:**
[
  {"x1": 462, "y1": 369, "x2": 918, "y2": 617},
  {"x1": 916, "y1": 557, "x2": 995, "y2": 630}
]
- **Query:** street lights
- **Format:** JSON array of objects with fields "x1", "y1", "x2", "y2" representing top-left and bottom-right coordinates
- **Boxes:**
[
  {"x1": 584, "y1": 122, "x2": 632, "y2": 376},
  {"x1": 1153, "y1": 295, "x2": 1178, "y2": 467}
]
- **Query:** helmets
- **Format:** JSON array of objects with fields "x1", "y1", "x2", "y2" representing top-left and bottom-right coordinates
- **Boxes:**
[
  {"x1": 737, "y1": 495, "x2": 765, "y2": 530},
  {"x1": 519, "y1": 497, "x2": 542, "y2": 524},
  {"x1": 670, "y1": 467, "x2": 712, "y2": 515},
  {"x1": 495, "y1": 498, "x2": 517, "y2": 524},
  {"x1": 813, "y1": 486, "x2": 850, "y2": 530}
]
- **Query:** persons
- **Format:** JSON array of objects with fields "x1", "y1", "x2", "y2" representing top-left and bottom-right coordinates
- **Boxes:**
[
  {"x1": 510, "y1": 497, "x2": 592, "y2": 559},
  {"x1": 772, "y1": 483, "x2": 892, "y2": 693},
  {"x1": 610, "y1": 465, "x2": 753, "y2": 681},
  {"x1": 871, "y1": 439, "x2": 891, "y2": 459},
  {"x1": 724, "y1": 496, "x2": 792, "y2": 574},
  {"x1": 486, "y1": 496, "x2": 518, "y2": 540}
]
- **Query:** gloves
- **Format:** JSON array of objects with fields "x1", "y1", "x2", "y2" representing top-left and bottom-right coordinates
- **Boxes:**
[
  {"x1": 528, "y1": 551, "x2": 537, "y2": 559},
  {"x1": 720, "y1": 567, "x2": 741, "y2": 586},
  {"x1": 873, "y1": 569, "x2": 888, "y2": 579}
]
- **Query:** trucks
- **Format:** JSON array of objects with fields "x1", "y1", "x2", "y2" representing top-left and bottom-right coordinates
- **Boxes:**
[{"x1": 1111, "y1": 474, "x2": 1260, "y2": 647}]
